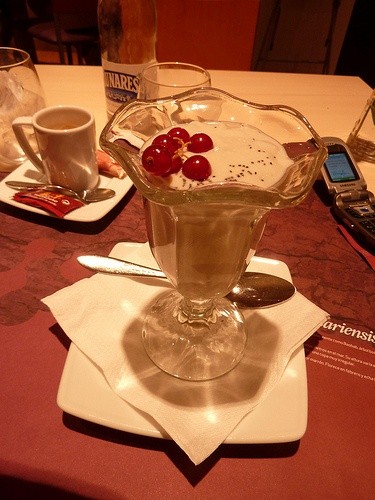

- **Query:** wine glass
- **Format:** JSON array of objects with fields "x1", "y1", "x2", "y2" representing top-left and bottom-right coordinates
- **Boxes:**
[{"x1": 99, "y1": 88, "x2": 326, "y2": 380}]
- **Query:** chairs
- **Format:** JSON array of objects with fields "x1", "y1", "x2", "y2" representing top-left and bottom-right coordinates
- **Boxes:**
[{"x1": 27, "y1": 0, "x2": 99, "y2": 66}]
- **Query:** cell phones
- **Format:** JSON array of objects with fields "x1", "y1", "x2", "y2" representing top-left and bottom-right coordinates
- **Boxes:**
[{"x1": 311, "y1": 136, "x2": 375, "y2": 253}]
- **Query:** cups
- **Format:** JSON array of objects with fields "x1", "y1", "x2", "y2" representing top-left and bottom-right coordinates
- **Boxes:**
[
  {"x1": 134, "y1": 62, "x2": 211, "y2": 103},
  {"x1": 0, "y1": 47, "x2": 44, "y2": 179},
  {"x1": 12, "y1": 106, "x2": 100, "y2": 195}
]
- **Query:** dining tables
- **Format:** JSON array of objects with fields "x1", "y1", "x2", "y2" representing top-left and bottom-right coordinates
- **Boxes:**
[{"x1": 0, "y1": 63, "x2": 375, "y2": 500}]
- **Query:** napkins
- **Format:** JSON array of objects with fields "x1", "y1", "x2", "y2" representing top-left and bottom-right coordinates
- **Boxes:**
[{"x1": 40, "y1": 242, "x2": 331, "y2": 465}]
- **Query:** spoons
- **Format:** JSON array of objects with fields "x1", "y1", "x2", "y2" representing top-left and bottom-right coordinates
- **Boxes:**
[
  {"x1": 77, "y1": 255, "x2": 295, "y2": 308},
  {"x1": 5, "y1": 180, "x2": 115, "y2": 201}
]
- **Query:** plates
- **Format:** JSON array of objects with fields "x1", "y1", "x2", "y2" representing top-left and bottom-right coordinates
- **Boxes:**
[{"x1": 0, "y1": 149, "x2": 135, "y2": 221}]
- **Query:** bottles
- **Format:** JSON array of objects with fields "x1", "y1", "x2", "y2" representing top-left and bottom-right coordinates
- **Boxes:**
[{"x1": 97, "y1": 0, "x2": 158, "y2": 121}]
